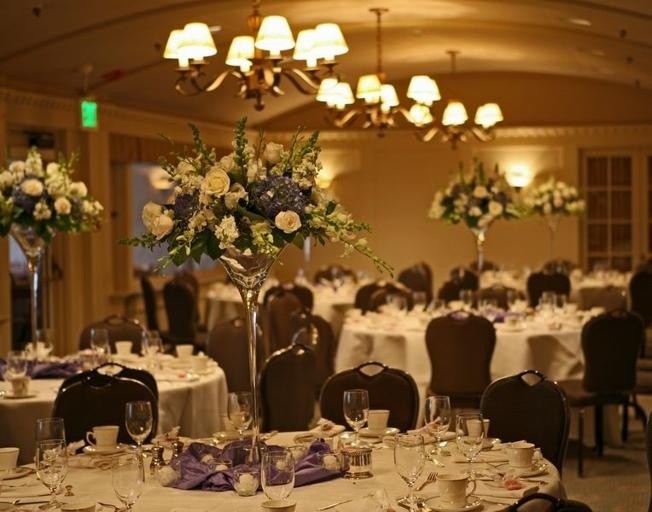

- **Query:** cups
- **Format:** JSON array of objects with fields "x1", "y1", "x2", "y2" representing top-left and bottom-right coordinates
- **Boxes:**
[
  {"x1": 341, "y1": 447, "x2": 374, "y2": 479},
  {"x1": 0, "y1": 447, "x2": 19, "y2": 472},
  {"x1": 322, "y1": 452, "x2": 343, "y2": 470},
  {"x1": 506, "y1": 443, "x2": 540, "y2": 467},
  {"x1": 7, "y1": 350, "x2": 28, "y2": 377},
  {"x1": 12, "y1": 377, "x2": 30, "y2": 395},
  {"x1": 36, "y1": 417, "x2": 67, "y2": 442},
  {"x1": 233, "y1": 470, "x2": 260, "y2": 495},
  {"x1": 207, "y1": 458, "x2": 233, "y2": 473},
  {"x1": 93, "y1": 346, "x2": 112, "y2": 366},
  {"x1": 87, "y1": 425, "x2": 120, "y2": 450},
  {"x1": 191, "y1": 356, "x2": 208, "y2": 371},
  {"x1": 176, "y1": 344, "x2": 194, "y2": 361},
  {"x1": 260, "y1": 499, "x2": 297, "y2": 511},
  {"x1": 243, "y1": 444, "x2": 259, "y2": 464},
  {"x1": 468, "y1": 419, "x2": 489, "y2": 441},
  {"x1": 436, "y1": 473, "x2": 476, "y2": 508},
  {"x1": 504, "y1": 317, "x2": 522, "y2": 330},
  {"x1": 157, "y1": 459, "x2": 182, "y2": 487},
  {"x1": 91, "y1": 329, "x2": 109, "y2": 353},
  {"x1": 592, "y1": 307, "x2": 605, "y2": 316},
  {"x1": 80, "y1": 354, "x2": 93, "y2": 371},
  {"x1": 367, "y1": 408, "x2": 389, "y2": 431},
  {"x1": 290, "y1": 445, "x2": 308, "y2": 463},
  {"x1": 115, "y1": 341, "x2": 133, "y2": 356},
  {"x1": 565, "y1": 304, "x2": 577, "y2": 312}
]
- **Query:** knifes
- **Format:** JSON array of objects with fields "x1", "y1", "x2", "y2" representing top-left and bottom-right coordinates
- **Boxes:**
[{"x1": 425, "y1": 450, "x2": 446, "y2": 467}]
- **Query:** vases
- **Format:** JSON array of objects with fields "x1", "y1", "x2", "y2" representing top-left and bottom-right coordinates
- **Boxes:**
[
  {"x1": 540, "y1": 213, "x2": 564, "y2": 274},
  {"x1": 470, "y1": 224, "x2": 490, "y2": 273},
  {"x1": 10, "y1": 226, "x2": 57, "y2": 350}
]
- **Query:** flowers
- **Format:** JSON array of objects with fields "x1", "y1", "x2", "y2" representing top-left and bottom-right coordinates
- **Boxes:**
[
  {"x1": 520, "y1": 168, "x2": 588, "y2": 217},
  {"x1": 0, "y1": 145, "x2": 105, "y2": 244},
  {"x1": 116, "y1": 116, "x2": 396, "y2": 279},
  {"x1": 425, "y1": 156, "x2": 524, "y2": 229}
]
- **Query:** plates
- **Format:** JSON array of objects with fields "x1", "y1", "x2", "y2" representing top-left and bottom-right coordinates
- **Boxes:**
[
  {"x1": 61, "y1": 501, "x2": 96, "y2": 510},
  {"x1": 495, "y1": 464, "x2": 545, "y2": 477},
  {"x1": 1, "y1": 468, "x2": 31, "y2": 479},
  {"x1": 359, "y1": 426, "x2": 401, "y2": 437},
  {"x1": 191, "y1": 366, "x2": 215, "y2": 375},
  {"x1": 83, "y1": 445, "x2": 121, "y2": 454},
  {"x1": 215, "y1": 430, "x2": 254, "y2": 440},
  {"x1": 497, "y1": 327, "x2": 527, "y2": 334},
  {"x1": 0, "y1": 501, "x2": 13, "y2": 510},
  {"x1": 484, "y1": 437, "x2": 501, "y2": 449},
  {"x1": 5, "y1": 389, "x2": 40, "y2": 398},
  {"x1": 169, "y1": 373, "x2": 192, "y2": 382},
  {"x1": 426, "y1": 497, "x2": 482, "y2": 511}
]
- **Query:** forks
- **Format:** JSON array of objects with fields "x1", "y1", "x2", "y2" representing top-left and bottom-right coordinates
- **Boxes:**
[{"x1": 416, "y1": 471, "x2": 437, "y2": 490}]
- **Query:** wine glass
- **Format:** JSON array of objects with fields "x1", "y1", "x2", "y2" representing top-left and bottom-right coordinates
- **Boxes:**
[
  {"x1": 37, "y1": 329, "x2": 55, "y2": 359},
  {"x1": 126, "y1": 402, "x2": 153, "y2": 454},
  {"x1": 145, "y1": 338, "x2": 160, "y2": 368},
  {"x1": 387, "y1": 289, "x2": 567, "y2": 331},
  {"x1": 293, "y1": 265, "x2": 370, "y2": 295},
  {"x1": 36, "y1": 439, "x2": 67, "y2": 510},
  {"x1": 425, "y1": 394, "x2": 453, "y2": 454},
  {"x1": 456, "y1": 413, "x2": 485, "y2": 479},
  {"x1": 342, "y1": 388, "x2": 369, "y2": 443},
  {"x1": 261, "y1": 450, "x2": 296, "y2": 499},
  {"x1": 112, "y1": 455, "x2": 144, "y2": 512},
  {"x1": 393, "y1": 434, "x2": 426, "y2": 511},
  {"x1": 227, "y1": 392, "x2": 254, "y2": 439},
  {"x1": 481, "y1": 259, "x2": 629, "y2": 287}
]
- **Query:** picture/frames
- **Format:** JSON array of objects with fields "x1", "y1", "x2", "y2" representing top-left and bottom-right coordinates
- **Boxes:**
[{"x1": 217, "y1": 245, "x2": 286, "y2": 458}]
